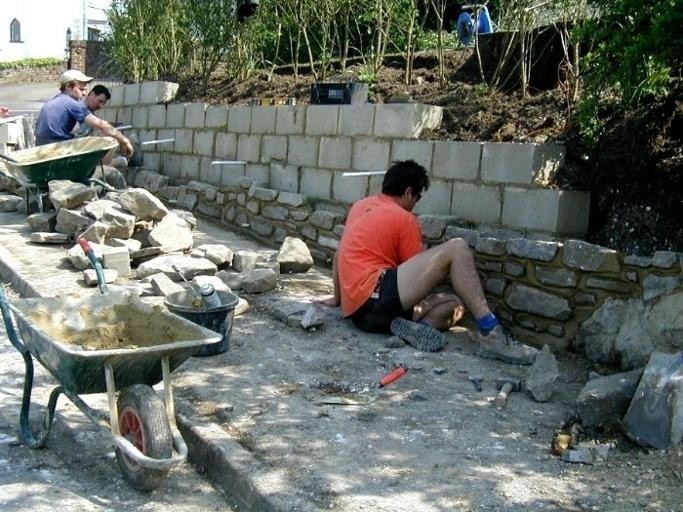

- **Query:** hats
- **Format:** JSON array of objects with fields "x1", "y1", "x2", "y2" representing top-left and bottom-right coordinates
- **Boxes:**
[{"x1": 59, "y1": 69, "x2": 93, "y2": 85}]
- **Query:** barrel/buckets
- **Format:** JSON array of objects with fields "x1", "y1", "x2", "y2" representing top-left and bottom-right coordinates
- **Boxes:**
[{"x1": 165, "y1": 265, "x2": 239, "y2": 356}]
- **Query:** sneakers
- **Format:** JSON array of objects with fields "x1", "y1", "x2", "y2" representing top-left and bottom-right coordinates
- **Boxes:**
[
  {"x1": 475, "y1": 321, "x2": 537, "y2": 365},
  {"x1": 390, "y1": 317, "x2": 447, "y2": 351}
]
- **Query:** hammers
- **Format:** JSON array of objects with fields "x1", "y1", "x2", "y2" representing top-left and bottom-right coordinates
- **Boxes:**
[{"x1": 495, "y1": 377, "x2": 521, "y2": 406}]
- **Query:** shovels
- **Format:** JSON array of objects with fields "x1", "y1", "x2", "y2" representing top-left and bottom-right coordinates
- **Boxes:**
[{"x1": 322, "y1": 366, "x2": 408, "y2": 405}]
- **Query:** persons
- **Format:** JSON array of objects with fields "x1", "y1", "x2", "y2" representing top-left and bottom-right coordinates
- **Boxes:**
[
  {"x1": 69, "y1": 85, "x2": 112, "y2": 138},
  {"x1": 33, "y1": 68, "x2": 136, "y2": 166},
  {"x1": 307, "y1": 157, "x2": 536, "y2": 366}
]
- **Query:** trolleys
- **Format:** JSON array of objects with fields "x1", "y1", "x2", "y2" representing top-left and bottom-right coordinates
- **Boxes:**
[
  {"x1": 0, "y1": 135, "x2": 120, "y2": 214},
  {"x1": 0, "y1": 238, "x2": 224, "y2": 493}
]
